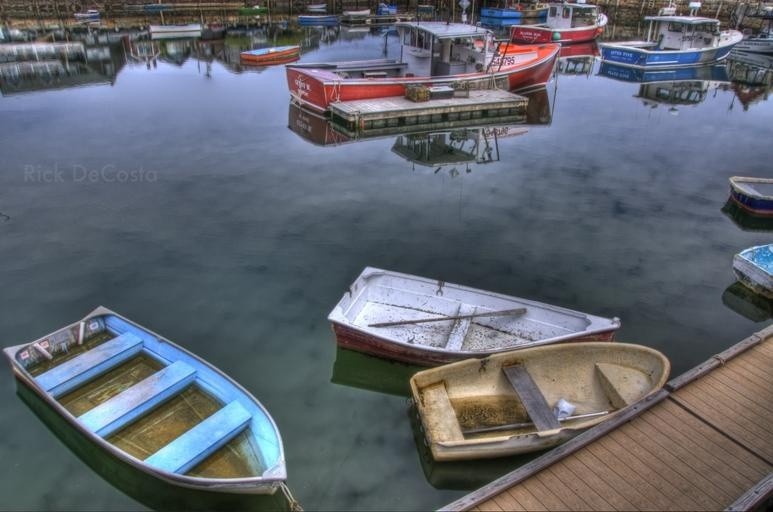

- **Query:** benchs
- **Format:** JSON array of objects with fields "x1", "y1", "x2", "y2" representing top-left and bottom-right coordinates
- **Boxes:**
[
  {"x1": 502, "y1": 365, "x2": 560, "y2": 431},
  {"x1": 32, "y1": 331, "x2": 197, "y2": 439},
  {"x1": 143, "y1": 401, "x2": 253, "y2": 477},
  {"x1": 420, "y1": 381, "x2": 465, "y2": 443}
]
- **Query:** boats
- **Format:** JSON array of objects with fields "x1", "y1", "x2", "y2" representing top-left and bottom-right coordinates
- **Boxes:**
[
  {"x1": 596, "y1": 14, "x2": 746, "y2": 70},
  {"x1": 507, "y1": 1, "x2": 610, "y2": 44},
  {"x1": 728, "y1": 171, "x2": 773, "y2": 299},
  {"x1": 1, "y1": 305, "x2": 288, "y2": 498},
  {"x1": 238, "y1": 44, "x2": 300, "y2": 63},
  {"x1": 284, "y1": 17, "x2": 567, "y2": 117}
]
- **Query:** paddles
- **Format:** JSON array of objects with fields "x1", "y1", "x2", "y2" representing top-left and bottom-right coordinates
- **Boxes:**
[
  {"x1": 367, "y1": 308, "x2": 526, "y2": 327},
  {"x1": 462, "y1": 411, "x2": 608, "y2": 435}
]
